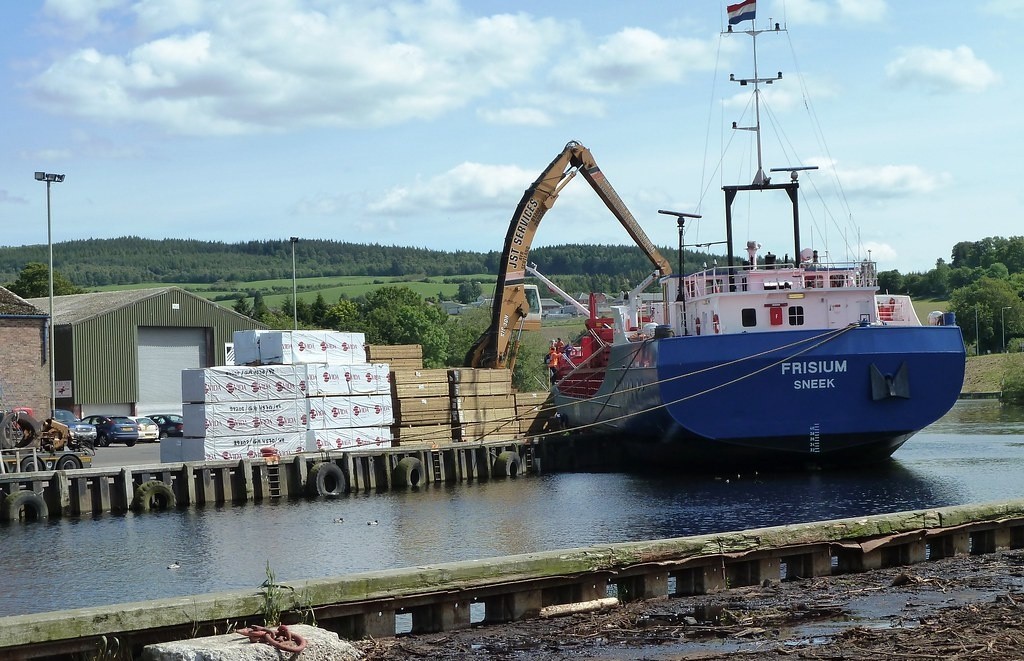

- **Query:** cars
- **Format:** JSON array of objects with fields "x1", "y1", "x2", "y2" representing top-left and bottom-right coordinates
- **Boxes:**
[
  {"x1": 145, "y1": 413, "x2": 183, "y2": 440},
  {"x1": 128, "y1": 415, "x2": 160, "y2": 443},
  {"x1": 81, "y1": 414, "x2": 139, "y2": 448}
]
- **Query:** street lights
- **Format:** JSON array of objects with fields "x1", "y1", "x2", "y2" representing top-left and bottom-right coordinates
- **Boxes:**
[
  {"x1": 1001, "y1": 306, "x2": 1013, "y2": 352},
  {"x1": 34, "y1": 170, "x2": 68, "y2": 418},
  {"x1": 289, "y1": 236, "x2": 300, "y2": 329}
]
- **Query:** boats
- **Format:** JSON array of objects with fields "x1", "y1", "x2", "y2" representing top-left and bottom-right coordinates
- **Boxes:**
[{"x1": 518, "y1": 1, "x2": 967, "y2": 476}]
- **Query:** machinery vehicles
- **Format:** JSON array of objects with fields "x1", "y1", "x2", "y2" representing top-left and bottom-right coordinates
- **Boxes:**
[{"x1": 459, "y1": 139, "x2": 673, "y2": 376}]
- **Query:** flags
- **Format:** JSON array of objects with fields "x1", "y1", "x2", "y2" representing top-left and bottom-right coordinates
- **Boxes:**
[{"x1": 727, "y1": 0, "x2": 756, "y2": 24}]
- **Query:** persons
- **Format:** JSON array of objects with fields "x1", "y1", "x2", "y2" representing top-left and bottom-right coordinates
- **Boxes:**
[{"x1": 548, "y1": 337, "x2": 564, "y2": 384}]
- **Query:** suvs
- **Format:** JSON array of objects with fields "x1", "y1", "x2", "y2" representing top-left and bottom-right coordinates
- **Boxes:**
[{"x1": 50, "y1": 408, "x2": 97, "y2": 444}]
